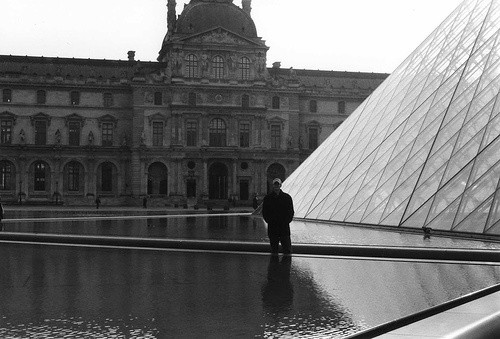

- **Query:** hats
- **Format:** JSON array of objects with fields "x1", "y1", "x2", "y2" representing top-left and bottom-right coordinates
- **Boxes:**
[{"x1": 273, "y1": 177, "x2": 282, "y2": 185}]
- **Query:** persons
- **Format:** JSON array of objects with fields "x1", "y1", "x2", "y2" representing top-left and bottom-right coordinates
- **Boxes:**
[
  {"x1": 262, "y1": 178, "x2": 294, "y2": 254},
  {"x1": 252, "y1": 196, "x2": 259, "y2": 210},
  {"x1": 96, "y1": 198, "x2": 101, "y2": 208}
]
